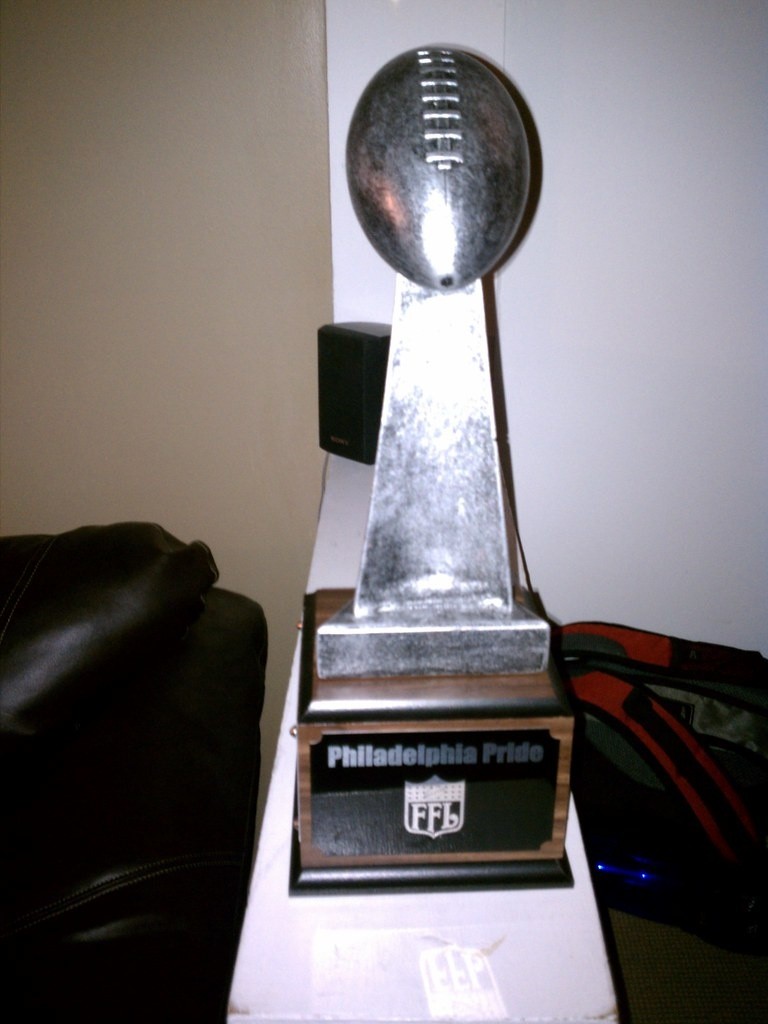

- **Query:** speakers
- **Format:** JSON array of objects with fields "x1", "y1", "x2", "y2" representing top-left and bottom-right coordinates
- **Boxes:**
[{"x1": 318, "y1": 320, "x2": 392, "y2": 466}]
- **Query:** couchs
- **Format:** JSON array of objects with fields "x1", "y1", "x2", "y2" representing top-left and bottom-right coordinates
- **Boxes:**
[{"x1": 0, "y1": 520, "x2": 269, "y2": 1024}]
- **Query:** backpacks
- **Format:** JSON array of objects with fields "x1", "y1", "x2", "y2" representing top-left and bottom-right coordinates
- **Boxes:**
[{"x1": 551, "y1": 621, "x2": 768, "y2": 957}]
